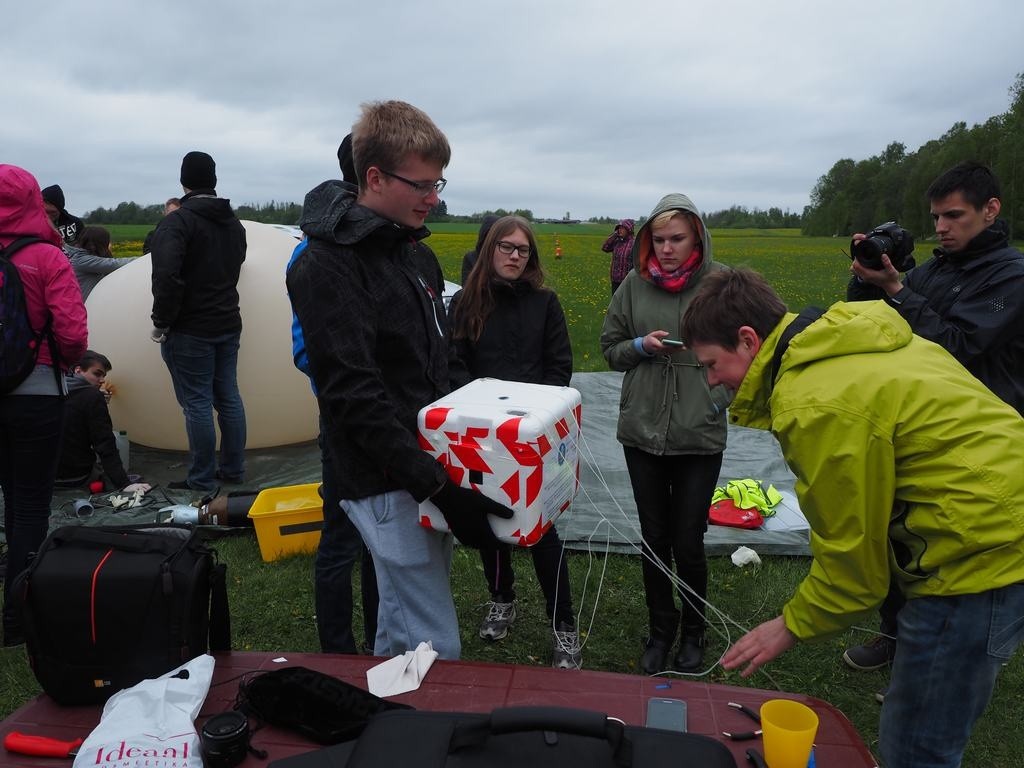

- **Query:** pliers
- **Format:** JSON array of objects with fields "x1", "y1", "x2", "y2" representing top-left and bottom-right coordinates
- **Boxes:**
[{"x1": 724, "y1": 700, "x2": 764, "y2": 740}]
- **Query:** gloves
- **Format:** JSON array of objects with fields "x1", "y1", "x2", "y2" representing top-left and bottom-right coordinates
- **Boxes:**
[{"x1": 430, "y1": 481, "x2": 513, "y2": 550}]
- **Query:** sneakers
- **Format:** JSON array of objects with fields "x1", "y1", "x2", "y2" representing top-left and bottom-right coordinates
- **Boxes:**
[
  {"x1": 479, "y1": 599, "x2": 516, "y2": 641},
  {"x1": 552, "y1": 629, "x2": 582, "y2": 672}
]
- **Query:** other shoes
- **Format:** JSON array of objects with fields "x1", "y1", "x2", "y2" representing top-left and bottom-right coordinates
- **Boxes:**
[
  {"x1": 215, "y1": 470, "x2": 242, "y2": 483},
  {"x1": 167, "y1": 480, "x2": 191, "y2": 489},
  {"x1": 843, "y1": 635, "x2": 896, "y2": 670}
]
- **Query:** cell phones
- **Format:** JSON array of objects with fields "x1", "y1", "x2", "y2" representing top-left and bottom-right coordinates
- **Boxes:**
[
  {"x1": 646, "y1": 696, "x2": 688, "y2": 734},
  {"x1": 662, "y1": 338, "x2": 683, "y2": 347}
]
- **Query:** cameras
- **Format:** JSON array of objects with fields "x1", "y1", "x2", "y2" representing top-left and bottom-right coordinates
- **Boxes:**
[{"x1": 854, "y1": 219, "x2": 917, "y2": 273}]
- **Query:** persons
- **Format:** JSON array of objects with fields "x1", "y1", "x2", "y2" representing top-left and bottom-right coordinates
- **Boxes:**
[
  {"x1": 449, "y1": 214, "x2": 584, "y2": 672},
  {"x1": 54, "y1": 349, "x2": 152, "y2": 495},
  {"x1": 601, "y1": 218, "x2": 636, "y2": 295},
  {"x1": 286, "y1": 132, "x2": 444, "y2": 656},
  {"x1": 286, "y1": 96, "x2": 514, "y2": 660},
  {"x1": 843, "y1": 159, "x2": 1024, "y2": 671},
  {"x1": 461, "y1": 214, "x2": 499, "y2": 290},
  {"x1": 142, "y1": 198, "x2": 181, "y2": 255},
  {"x1": 40, "y1": 183, "x2": 86, "y2": 247},
  {"x1": 679, "y1": 264, "x2": 1024, "y2": 768},
  {"x1": 61, "y1": 226, "x2": 141, "y2": 303},
  {"x1": 148, "y1": 151, "x2": 247, "y2": 492},
  {"x1": 0, "y1": 161, "x2": 89, "y2": 653},
  {"x1": 601, "y1": 191, "x2": 731, "y2": 673}
]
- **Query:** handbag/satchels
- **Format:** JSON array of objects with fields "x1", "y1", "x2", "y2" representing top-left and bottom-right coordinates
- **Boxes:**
[{"x1": 8, "y1": 519, "x2": 231, "y2": 710}]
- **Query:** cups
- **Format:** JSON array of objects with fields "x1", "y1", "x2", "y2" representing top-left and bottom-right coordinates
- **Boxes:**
[{"x1": 760, "y1": 699, "x2": 819, "y2": 768}]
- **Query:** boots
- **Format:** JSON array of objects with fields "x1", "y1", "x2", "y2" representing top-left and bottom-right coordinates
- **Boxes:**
[
  {"x1": 674, "y1": 606, "x2": 708, "y2": 673},
  {"x1": 639, "y1": 608, "x2": 681, "y2": 674}
]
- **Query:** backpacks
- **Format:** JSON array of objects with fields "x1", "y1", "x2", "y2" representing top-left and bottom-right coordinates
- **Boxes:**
[{"x1": 0, "y1": 235, "x2": 61, "y2": 396}]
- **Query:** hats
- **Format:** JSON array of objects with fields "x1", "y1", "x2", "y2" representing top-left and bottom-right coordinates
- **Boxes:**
[
  {"x1": 180, "y1": 151, "x2": 217, "y2": 190},
  {"x1": 337, "y1": 133, "x2": 358, "y2": 186},
  {"x1": 41, "y1": 185, "x2": 64, "y2": 209}
]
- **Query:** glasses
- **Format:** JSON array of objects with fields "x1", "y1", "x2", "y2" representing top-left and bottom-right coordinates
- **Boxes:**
[
  {"x1": 370, "y1": 166, "x2": 447, "y2": 194},
  {"x1": 496, "y1": 241, "x2": 533, "y2": 258}
]
